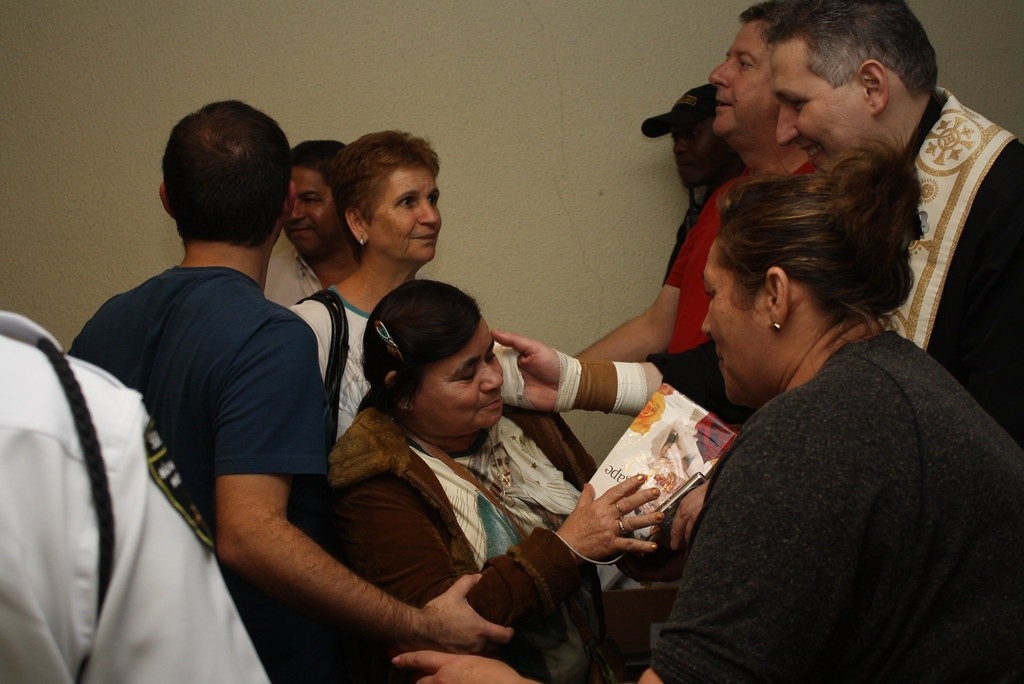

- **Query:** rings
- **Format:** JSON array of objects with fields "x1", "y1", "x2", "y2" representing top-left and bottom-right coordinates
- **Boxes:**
[
  {"x1": 618, "y1": 519, "x2": 625, "y2": 534},
  {"x1": 615, "y1": 503, "x2": 624, "y2": 516}
]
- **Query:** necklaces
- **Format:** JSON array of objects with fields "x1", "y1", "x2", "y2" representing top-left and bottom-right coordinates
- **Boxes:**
[{"x1": 486, "y1": 431, "x2": 508, "y2": 502}]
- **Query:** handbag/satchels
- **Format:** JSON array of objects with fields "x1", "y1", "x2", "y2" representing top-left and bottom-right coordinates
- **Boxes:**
[{"x1": 586, "y1": 634, "x2": 626, "y2": 684}]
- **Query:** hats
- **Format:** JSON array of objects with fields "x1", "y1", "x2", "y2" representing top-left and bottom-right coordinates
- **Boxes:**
[{"x1": 641, "y1": 83, "x2": 719, "y2": 138}]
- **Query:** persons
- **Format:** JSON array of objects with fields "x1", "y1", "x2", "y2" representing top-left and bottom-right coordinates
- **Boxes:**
[
  {"x1": 393, "y1": 0, "x2": 1024, "y2": 684},
  {"x1": 0, "y1": 98, "x2": 664, "y2": 684}
]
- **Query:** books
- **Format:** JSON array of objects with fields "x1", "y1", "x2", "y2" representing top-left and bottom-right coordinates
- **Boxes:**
[
  {"x1": 590, "y1": 381, "x2": 735, "y2": 555},
  {"x1": 656, "y1": 472, "x2": 708, "y2": 534}
]
